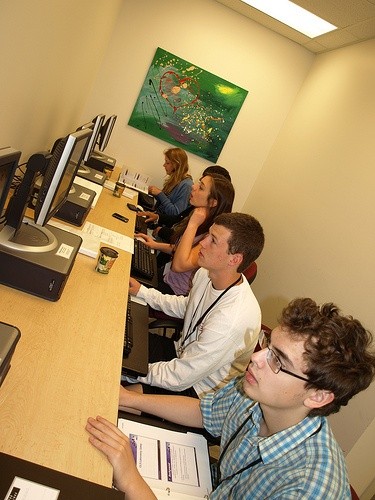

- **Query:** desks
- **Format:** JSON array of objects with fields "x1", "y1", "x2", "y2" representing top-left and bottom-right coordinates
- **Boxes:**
[{"x1": 0, "y1": 166, "x2": 138, "y2": 500}]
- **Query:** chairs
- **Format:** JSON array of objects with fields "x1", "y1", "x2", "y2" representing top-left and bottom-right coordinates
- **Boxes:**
[{"x1": 148, "y1": 263, "x2": 362, "y2": 500}]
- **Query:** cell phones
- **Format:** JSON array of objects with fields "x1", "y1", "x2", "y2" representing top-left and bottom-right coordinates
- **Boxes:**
[
  {"x1": 127, "y1": 203, "x2": 137, "y2": 210},
  {"x1": 112, "y1": 212, "x2": 129, "y2": 223}
]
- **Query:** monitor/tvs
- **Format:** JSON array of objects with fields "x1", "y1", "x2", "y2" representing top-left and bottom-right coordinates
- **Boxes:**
[
  {"x1": 0, "y1": 144, "x2": 21, "y2": 214},
  {"x1": 78, "y1": 113, "x2": 105, "y2": 162},
  {"x1": 0, "y1": 127, "x2": 93, "y2": 242},
  {"x1": 96, "y1": 113, "x2": 117, "y2": 151}
]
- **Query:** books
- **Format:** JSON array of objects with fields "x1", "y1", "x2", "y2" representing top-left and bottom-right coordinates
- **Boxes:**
[
  {"x1": 47, "y1": 219, "x2": 101, "y2": 259},
  {"x1": 118, "y1": 167, "x2": 151, "y2": 195}
]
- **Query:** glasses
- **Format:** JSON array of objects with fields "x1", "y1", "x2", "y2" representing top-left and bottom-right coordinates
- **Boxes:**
[{"x1": 259, "y1": 329, "x2": 320, "y2": 388}]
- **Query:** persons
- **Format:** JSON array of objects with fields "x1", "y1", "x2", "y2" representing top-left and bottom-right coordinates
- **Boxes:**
[
  {"x1": 118, "y1": 213, "x2": 262, "y2": 423},
  {"x1": 85, "y1": 298, "x2": 375, "y2": 500},
  {"x1": 133, "y1": 147, "x2": 235, "y2": 309}
]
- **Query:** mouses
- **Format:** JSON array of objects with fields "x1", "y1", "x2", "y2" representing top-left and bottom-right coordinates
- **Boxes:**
[{"x1": 134, "y1": 236, "x2": 146, "y2": 242}]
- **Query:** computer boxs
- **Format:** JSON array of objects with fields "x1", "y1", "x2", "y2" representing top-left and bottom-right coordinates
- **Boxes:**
[
  {"x1": 0, "y1": 211, "x2": 83, "y2": 302},
  {"x1": 71, "y1": 149, "x2": 116, "y2": 210},
  {"x1": 29, "y1": 176, "x2": 97, "y2": 227}
]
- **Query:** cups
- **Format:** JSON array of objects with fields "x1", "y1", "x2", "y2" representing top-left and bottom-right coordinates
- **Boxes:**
[
  {"x1": 94, "y1": 247, "x2": 119, "y2": 274},
  {"x1": 113, "y1": 182, "x2": 126, "y2": 198}
]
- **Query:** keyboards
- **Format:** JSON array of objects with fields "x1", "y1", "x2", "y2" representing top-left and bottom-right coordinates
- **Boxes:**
[
  {"x1": 134, "y1": 215, "x2": 147, "y2": 235},
  {"x1": 123, "y1": 291, "x2": 134, "y2": 359},
  {"x1": 131, "y1": 238, "x2": 154, "y2": 280}
]
